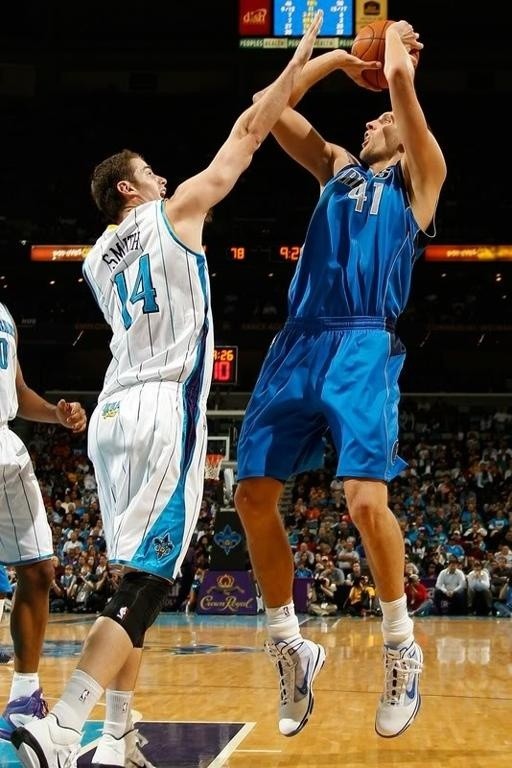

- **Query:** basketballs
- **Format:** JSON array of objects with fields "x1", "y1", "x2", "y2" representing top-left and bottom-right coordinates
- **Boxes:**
[{"x1": 352, "y1": 20, "x2": 420, "y2": 89}]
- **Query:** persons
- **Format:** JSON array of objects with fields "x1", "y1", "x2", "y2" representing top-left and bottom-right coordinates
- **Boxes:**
[
  {"x1": 0, "y1": 401, "x2": 512, "y2": 622},
  {"x1": 0, "y1": 301, "x2": 87, "y2": 741},
  {"x1": 11, "y1": 9, "x2": 324, "y2": 768},
  {"x1": 233, "y1": 17, "x2": 447, "y2": 738}
]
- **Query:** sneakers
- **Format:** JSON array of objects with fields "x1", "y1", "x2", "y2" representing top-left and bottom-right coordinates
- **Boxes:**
[
  {"x1": 375, "y1": 640, "x2": 422, "y2": 739},
  {"x1": 263, "y1": 639, "x2": 326, "y2": 737},
  {"x1": 0, "y1": 688, "x2": 155, "y2": 768}
]
